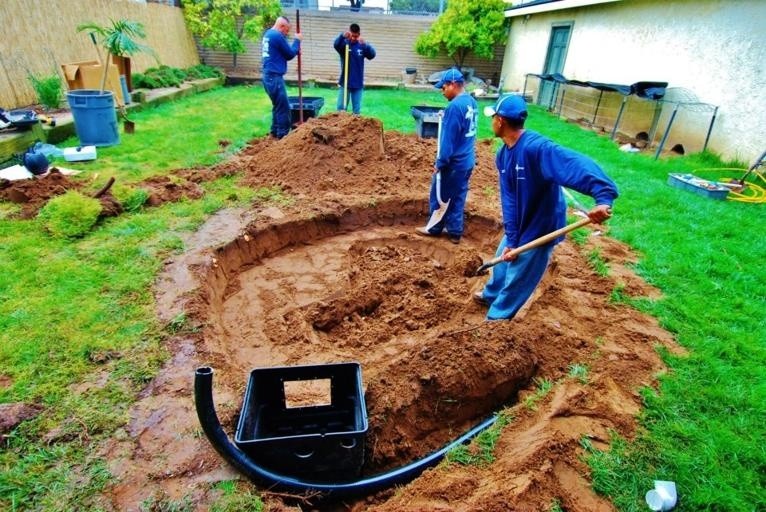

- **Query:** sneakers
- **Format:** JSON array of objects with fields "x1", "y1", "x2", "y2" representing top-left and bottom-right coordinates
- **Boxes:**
[
  {"x1": 415, "y1": 226, "x2": 429, "y2": 235},
  {"x1": 473, "y1": 291, "x2": 488, "y2": 305}
]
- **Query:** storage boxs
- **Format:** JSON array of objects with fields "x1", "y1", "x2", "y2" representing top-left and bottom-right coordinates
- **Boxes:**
[
  {"x1": 61, "y1": 59, "x2": 125, "y2": 108},
  {"x1": 668, "y1": 169, "x2": 730, "y2": 201}
]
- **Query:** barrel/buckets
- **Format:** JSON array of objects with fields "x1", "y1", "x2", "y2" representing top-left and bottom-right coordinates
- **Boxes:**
[{"x1": 402, "y1": 71, "x2": 416, "y2": 84}]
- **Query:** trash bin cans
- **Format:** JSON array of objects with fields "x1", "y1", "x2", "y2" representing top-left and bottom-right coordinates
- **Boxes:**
[
  {"x1": 234, "y1": 360, "x2": 368, "y2": 480},
  {"x1": 287, "y1": 96, "x2": 324, "y2": 130},
  {"x1": 406, "y1": 68, "x2": 417, "y2": 84},
  {"x1": 411, "y1": 106, "x2": 447, "y2": 138}
]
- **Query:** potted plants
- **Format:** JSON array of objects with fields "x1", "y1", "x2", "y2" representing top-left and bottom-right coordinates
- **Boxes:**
[{"x1": 76, "y1": 16, "x2": 160, "y2": 93}]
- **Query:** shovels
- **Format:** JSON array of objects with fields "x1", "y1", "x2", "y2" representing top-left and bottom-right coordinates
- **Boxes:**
[
  {"x1": 90, "y1": 32, "x2": 134, "y2": 134},
  {"x1": 454, "y1": 209, "x2": 612, "y2": 277},
  {"x1": 728, "y1": 151, "x2": 766, "y2": 193},
  {"x1": 426, "y1": 110, "x2": 451, "y2": 231}
]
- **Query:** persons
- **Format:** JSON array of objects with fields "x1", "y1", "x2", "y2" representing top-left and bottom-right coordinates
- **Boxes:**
[
  {"x1": 415, "y1": 68, "x2": 479, "y2": 243},
  {"x1": 261, "y1": 15, "x2": 303, "y2": 139},
  {"x1": 333, "y1": 24, "x2": 376, "y2": 118},
  {"x1": 474, "y1": 95, "x2": 618, "y2": 328}
]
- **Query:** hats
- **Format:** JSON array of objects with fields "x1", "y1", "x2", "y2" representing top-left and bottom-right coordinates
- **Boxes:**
[
  {"x1": 435, "y1": 68, "x2": 464, "y2": 88},
  {"x1": 483, "y1": 94, "x2": 528, "y2": 121}
]
